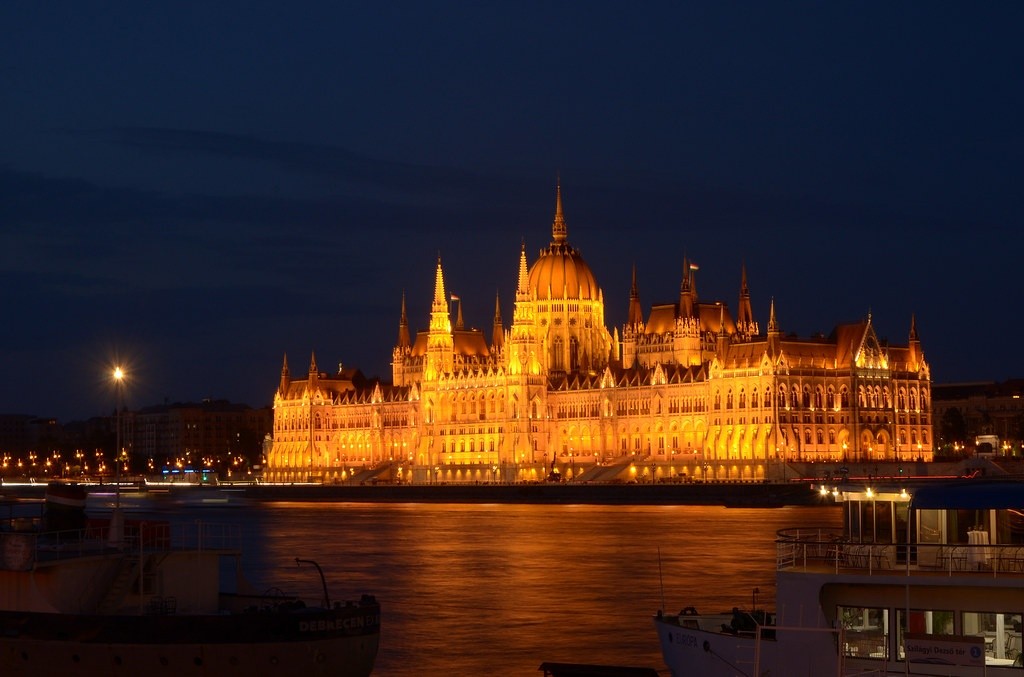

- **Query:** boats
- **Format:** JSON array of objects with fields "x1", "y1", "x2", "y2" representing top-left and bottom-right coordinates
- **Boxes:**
[
  {"x1": 654, "y1": 475, "x2": 1024, "y2": 677},
  {"x1": 1, "y1": 370, "x2": 380, "y2": 677}
]
